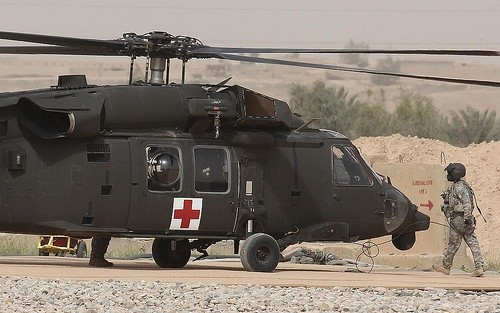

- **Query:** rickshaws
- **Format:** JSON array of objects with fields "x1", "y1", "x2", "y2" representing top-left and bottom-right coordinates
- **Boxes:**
[{"x1": 37, "y1": 234, "x2": 88, "y2": 258}]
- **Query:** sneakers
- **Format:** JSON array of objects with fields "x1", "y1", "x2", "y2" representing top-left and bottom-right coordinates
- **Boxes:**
[
  {"x1": 471, "y1": 268, "x2": 484, "y2": 277},
  {"x1": 432, "y1": 263, "x2": 450, "y2": 275}
]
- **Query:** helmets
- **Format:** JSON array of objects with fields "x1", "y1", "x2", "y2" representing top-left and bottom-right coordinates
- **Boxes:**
[{"x1": 444, "y1": 162, "x2": 466, "y2": 181}]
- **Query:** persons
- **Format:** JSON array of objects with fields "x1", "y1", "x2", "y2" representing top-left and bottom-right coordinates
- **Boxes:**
[
  {"x1": 432, "y1": 163, "x2": 485, "y2": 277},
  {"x1": 89, "y1": 236, "x2": 114, "y2": 267}
]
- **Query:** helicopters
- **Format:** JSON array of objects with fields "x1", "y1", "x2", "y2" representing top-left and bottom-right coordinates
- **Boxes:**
[{"x1": 0, "y1": 27, "x2": 500, "y2": 272}]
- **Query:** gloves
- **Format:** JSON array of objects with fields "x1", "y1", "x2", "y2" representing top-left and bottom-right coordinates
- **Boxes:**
[{"x1": 463, "y1": 219, "x2": 472, "y2": 230}]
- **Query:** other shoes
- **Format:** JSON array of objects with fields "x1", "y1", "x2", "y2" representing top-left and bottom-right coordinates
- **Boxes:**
[{"x1": 89, "y1": 257, "x2": 113, "y2": 267}]
